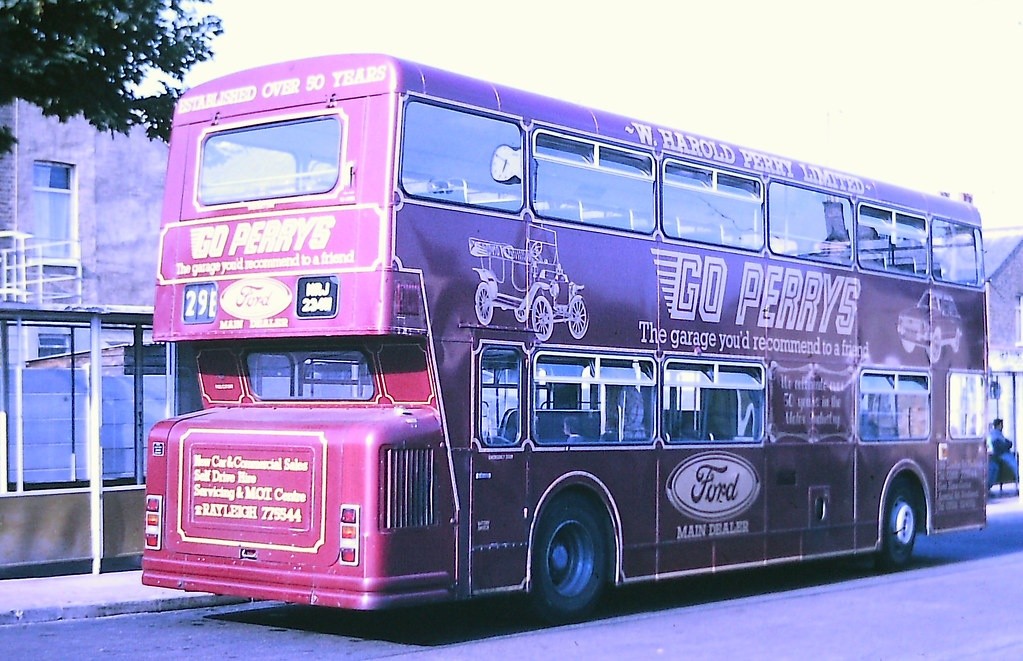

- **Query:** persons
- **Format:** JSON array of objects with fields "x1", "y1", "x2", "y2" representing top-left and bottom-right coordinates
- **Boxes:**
[
  {"x1": 563, "y1": 418, "x2": 582, "y2": 441},
  {"x1": 991, "y1": 418, "x2": 1019, "y2": 478},
  {"x1": 614, "y1": 385, "x2": 646, "y2": 432}
]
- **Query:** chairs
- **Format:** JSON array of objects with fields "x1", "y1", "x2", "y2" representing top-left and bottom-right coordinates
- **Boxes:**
[
  {"x1": 406, "y1": 174, "x2": 977, "y2": 283},
  {"x1": 481, "y1": 397, "x2": 765, "y2": 445}
]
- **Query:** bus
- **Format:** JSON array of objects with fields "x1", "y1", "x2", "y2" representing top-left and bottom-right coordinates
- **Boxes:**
[{"x1": 137, "y1": 52, "x2": 1003, "y2": 623}]
